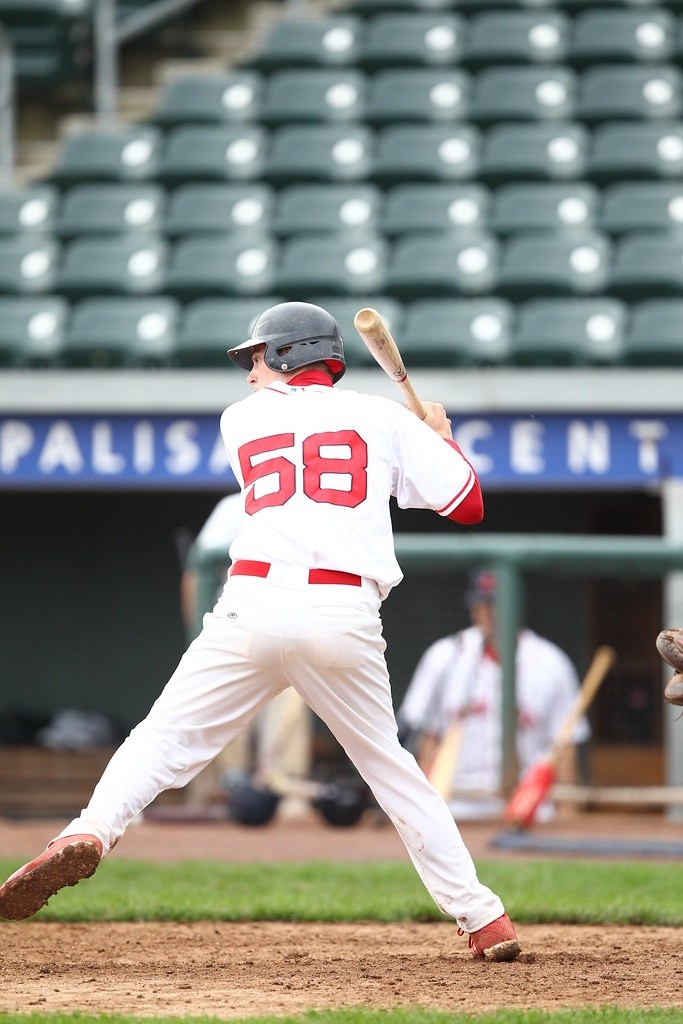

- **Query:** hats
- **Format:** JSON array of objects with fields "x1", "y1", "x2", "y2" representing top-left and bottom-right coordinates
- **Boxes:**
[{"x1": 464, "y1": 567, "x2": 496, "y2": 604}]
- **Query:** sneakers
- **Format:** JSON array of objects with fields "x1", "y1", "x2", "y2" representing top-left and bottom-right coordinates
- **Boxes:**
[
  {"x1": 1, "y1": 833, "x2": 103, "y2": 921},
  {"x1": 468, "y1": 913, "x2": 522, "y2": 962}
]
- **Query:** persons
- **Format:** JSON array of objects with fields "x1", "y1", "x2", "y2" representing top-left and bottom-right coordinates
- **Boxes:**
[
  {"x1": 400, "y1": 566, "x2": 589, "y2": 823},
  {"x1": 178, "y1": 489, "x2": 318, "y2": 823},
  {"x1": 0, "y1": 301, "x2": 519, "y2": 958}
]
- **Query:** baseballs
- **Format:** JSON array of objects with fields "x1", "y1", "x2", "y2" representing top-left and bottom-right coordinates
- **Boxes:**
[
  {"x1": 507, "y1": 642, "x2": 615, "y2": 832},
  {"x1": 352, "y1": 304, "x2": 425, "y2": 419}
]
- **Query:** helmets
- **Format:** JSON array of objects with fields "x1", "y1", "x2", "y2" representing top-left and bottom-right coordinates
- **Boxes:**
[{"x1": 227, "y1": 300, "x2": 347, "y2": 384}]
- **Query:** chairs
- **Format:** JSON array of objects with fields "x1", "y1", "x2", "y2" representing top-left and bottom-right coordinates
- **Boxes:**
[{"x1": 0, "y1": 15, "x2": 682, "y2": 371}]
